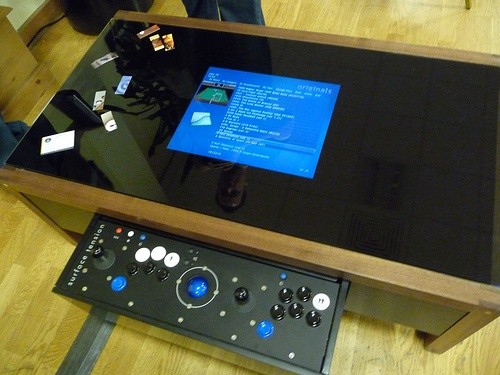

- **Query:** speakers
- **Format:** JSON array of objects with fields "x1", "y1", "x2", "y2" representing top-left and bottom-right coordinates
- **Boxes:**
[{"x1": 60, "y1": 1, "x2": 154, "y2": 38}]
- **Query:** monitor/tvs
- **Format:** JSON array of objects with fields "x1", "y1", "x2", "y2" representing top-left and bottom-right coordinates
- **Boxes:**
[{"x1": 166, "y1": 66, "x2": 341, "y2": 182}]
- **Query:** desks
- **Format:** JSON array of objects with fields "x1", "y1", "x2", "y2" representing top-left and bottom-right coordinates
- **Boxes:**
[{"x1": 0, "y1": 9, "x2": 500, "y2": 354}]
